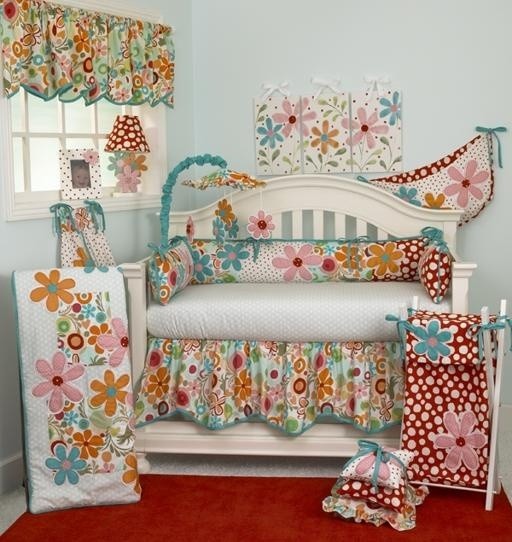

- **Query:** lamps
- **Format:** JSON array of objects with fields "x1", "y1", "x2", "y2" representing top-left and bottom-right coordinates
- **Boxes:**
[{"x1": 103, "y1": 114, "x2": 151, "y2": 192}]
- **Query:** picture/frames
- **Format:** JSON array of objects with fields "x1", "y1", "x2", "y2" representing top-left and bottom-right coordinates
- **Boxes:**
[{"x1": 60, "y1": 148, "x2": 102, "y2": 200}]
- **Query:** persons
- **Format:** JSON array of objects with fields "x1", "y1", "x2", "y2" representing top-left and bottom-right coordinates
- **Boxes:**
[{"x1": 71, "y1": 160, "x2": 91, "y2": 188}]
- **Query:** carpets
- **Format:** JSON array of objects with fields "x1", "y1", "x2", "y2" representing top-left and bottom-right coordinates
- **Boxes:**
[{"x1": 0, "y1": 473, "x2": 511, "y2": 542}]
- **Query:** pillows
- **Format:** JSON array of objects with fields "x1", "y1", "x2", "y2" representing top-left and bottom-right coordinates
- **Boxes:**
[
  {"x1": 323, "y1": 445, "x2": 426, "y2": 531},
  {"x1": 419, "y1": 227, "x2": 453, "y2": 304},
  {"x1": 147, "y1": 234, "x2": 194, "y2": 306}
]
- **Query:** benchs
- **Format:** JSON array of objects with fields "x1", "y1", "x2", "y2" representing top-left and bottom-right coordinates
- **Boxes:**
[{"x1": 118, "y1": 174, "x2": 479, "y2": 432}]
